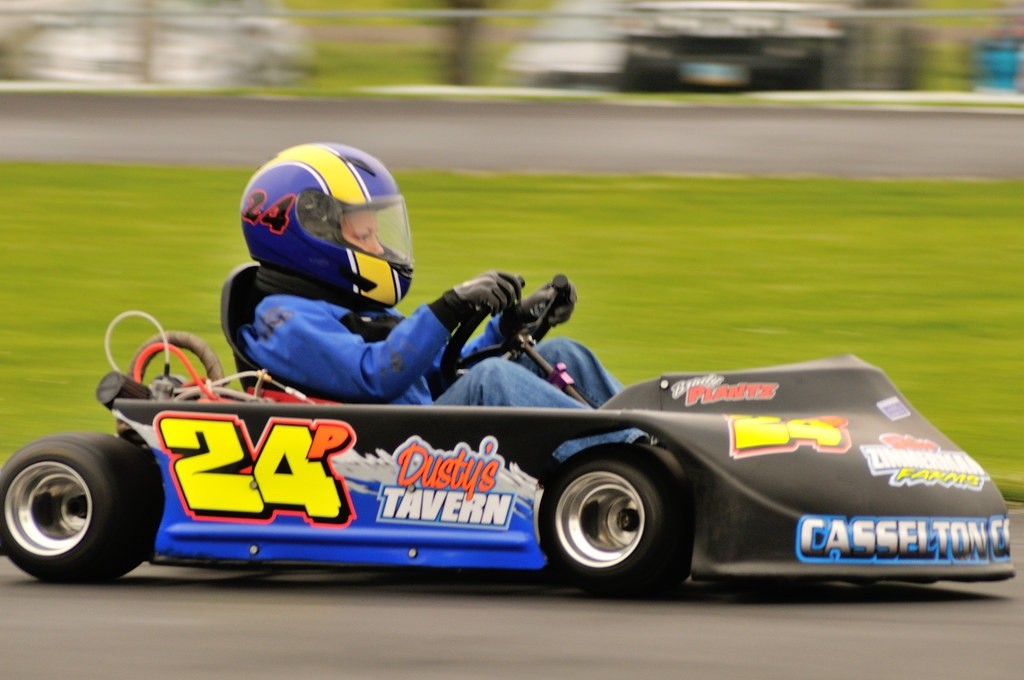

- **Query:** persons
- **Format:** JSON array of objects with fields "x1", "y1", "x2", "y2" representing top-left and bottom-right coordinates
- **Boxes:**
[{"x1": 242, "y1": 142, "x2": 627, "y2": 410}]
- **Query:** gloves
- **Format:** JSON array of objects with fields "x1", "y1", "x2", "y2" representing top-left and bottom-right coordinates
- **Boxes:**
[
  {"x1": 496, "y1": 281, "x2": 577, "y2": 340},
  {"x1": 442, "y1": 269, "x2": 522, "y2": 319}
]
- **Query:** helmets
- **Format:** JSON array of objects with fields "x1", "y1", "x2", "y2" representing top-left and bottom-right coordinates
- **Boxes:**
[{"x1": 240, "y1": 143, "x2": 415, "y2": 308}]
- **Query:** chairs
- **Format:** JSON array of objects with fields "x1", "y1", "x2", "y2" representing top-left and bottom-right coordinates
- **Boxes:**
[{"x1": 220, "y1": 259, "x2": 297, "y2": 393}]
